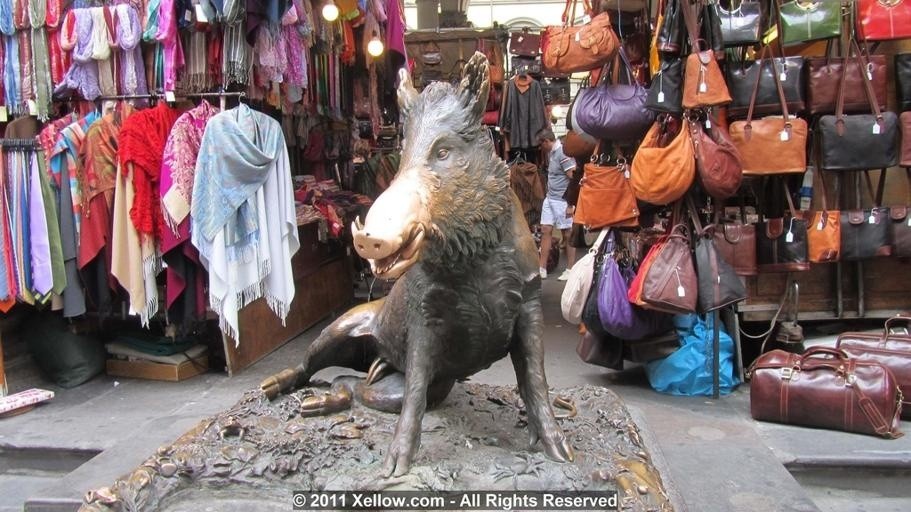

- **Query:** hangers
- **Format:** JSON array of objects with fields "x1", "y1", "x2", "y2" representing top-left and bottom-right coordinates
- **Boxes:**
[
  {"x1": 508, "y1": 65, "x2": 527, "y2": 78},
  {"x1": 67, "y1": 90, "x2": 246, "y2": 115},
  {"x1": 511, "y1": 150, "x2": 525, "y2": 165}
]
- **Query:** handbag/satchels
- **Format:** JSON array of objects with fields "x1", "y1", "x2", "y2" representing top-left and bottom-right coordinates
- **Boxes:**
[
  {"x1": 487, "y1": 46, "x2": 505, "y2": 85},
  {"x1": 510, "y1": 30, "x2": 540, "y2": 58},
  {"x1": 839, "y1": 312, "x2": 910, "y2": 422},
  {"x1": 510, "y1": 55, "x2": 540, "y2": 80},
  {"x1": 542, "y1": 0, "x2": 911, "y2": 372},
  {"x1": 751, "y1": 345, "x2": 906, "y2": 442},
  {"x1": 422, "y1": 52, "x2": 442, "y2": 65}
]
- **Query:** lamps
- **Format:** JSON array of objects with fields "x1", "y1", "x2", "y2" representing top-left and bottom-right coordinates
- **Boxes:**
[
  {"x1": 367, "y1": 30, "x2": 384, "y2": 56},
  {"x1": 321, "y1": 0, "x2": 338, "y2": 21}
]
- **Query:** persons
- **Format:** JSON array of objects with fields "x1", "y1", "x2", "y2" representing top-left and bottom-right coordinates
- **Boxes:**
[{"x1": 534, "y1": 127, "x2": 578, "y2": 282}]
